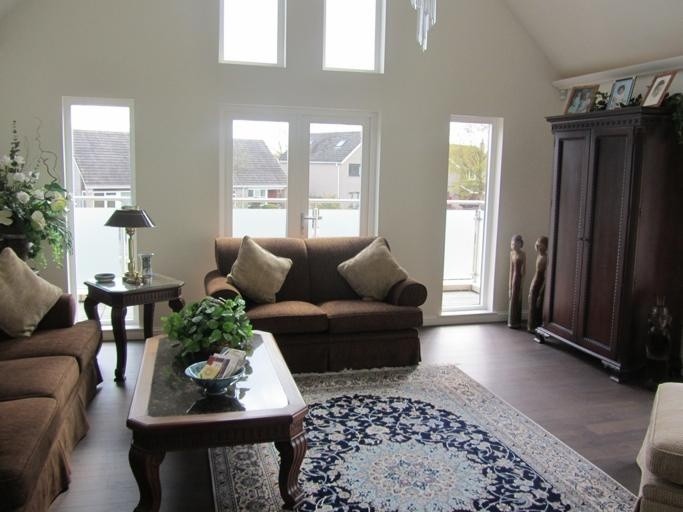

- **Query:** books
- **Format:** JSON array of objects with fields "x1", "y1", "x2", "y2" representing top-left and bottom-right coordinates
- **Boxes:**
[{"x1": 198, "y1": 347, "x2": 247, "y2": 379}]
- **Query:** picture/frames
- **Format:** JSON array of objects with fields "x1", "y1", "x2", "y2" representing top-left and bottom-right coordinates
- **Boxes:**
[
  {"x1": 640, "y1": 70, "x2": 677, "y2": 108},
  {"x1": 605, "y1": 76, "x2": 637, "y2": 111},
  {"x1": 561, "y1": 84, "x2": 599, "y2": 116}
]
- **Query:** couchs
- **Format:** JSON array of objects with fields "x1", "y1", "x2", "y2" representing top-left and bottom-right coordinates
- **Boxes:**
[
  {"x1": 0, "y1": 293, "x2": 102, "y2": 512},
  {"x1": 635, "y1": 382, "x2": 682, "y2": 512},
  {"x1": 203, "y1": 236, "x2": 427, "y2": 371}
]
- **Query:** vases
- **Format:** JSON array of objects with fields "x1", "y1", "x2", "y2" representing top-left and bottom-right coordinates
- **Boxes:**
[{"x1": 1, "y1": 233, "x2": 27, "y2": 263}]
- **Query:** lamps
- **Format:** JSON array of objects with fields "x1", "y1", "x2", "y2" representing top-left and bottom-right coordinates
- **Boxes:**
[{"x1": 104, "y1": 205, "x2": 156, "y2": 283}]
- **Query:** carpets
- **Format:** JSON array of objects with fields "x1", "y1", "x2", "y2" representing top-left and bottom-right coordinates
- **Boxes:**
[{"x1": 208, "y1": 364, "x2": 641, "y2": 511}]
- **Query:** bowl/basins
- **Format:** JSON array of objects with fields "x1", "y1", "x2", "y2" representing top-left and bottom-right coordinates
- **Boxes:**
[
  {"x1": 184, "y1": 360, "x2": 245, "y2": 396},
  {"x1": 94, "y1": 273, "x2": 115, "y2": 283}
]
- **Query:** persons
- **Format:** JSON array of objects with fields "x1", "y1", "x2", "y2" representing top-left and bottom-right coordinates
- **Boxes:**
[
  {"x1": 508, "y1": 235, "x2": 526, "y2": 328},
  {"x1": 642, "y1": 293, "x2": 672, "y2": 391},
  {"x1": 644, "y1": 80, "x2": 665, "y2": 105},
  {"x1": 527, "y1": 236, "x2": 548, "y2": 332},
  {"x1": 567, "y1": 89, "x2": 591, "y2": 113},
  {"x1": 612, "y1": 85, "x2": 625, "y2": 108}
]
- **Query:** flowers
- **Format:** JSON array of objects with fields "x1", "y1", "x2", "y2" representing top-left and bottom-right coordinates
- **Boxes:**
[{"x1": 0, "y1": 117, "x2": 73, "y2": 275}]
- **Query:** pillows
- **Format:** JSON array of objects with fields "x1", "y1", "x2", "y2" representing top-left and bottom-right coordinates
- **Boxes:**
[
  {"x1": 225, "y1": 235, "x2": 293, "y2": 304},
  {"x1": 0, "y1": 247, "x2": 65, "y2": 337},
  {"x1": 337, "y1": 237, "x2": 408, "y2": 303}
]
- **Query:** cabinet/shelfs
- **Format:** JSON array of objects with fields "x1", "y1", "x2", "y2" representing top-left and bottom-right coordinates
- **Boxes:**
[{"x1": 533, "y1": 103, "x2": 682, "y2": 384}]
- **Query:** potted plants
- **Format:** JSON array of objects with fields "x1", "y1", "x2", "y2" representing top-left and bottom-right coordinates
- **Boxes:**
[{"x1": 160, "y1": 295, "x2": 254, "y2": 372}]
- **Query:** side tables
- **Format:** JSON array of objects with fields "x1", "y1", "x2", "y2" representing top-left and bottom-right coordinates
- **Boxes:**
[{"x1": 83, "y1": 272, "x2": 186, "y2": 386}]
